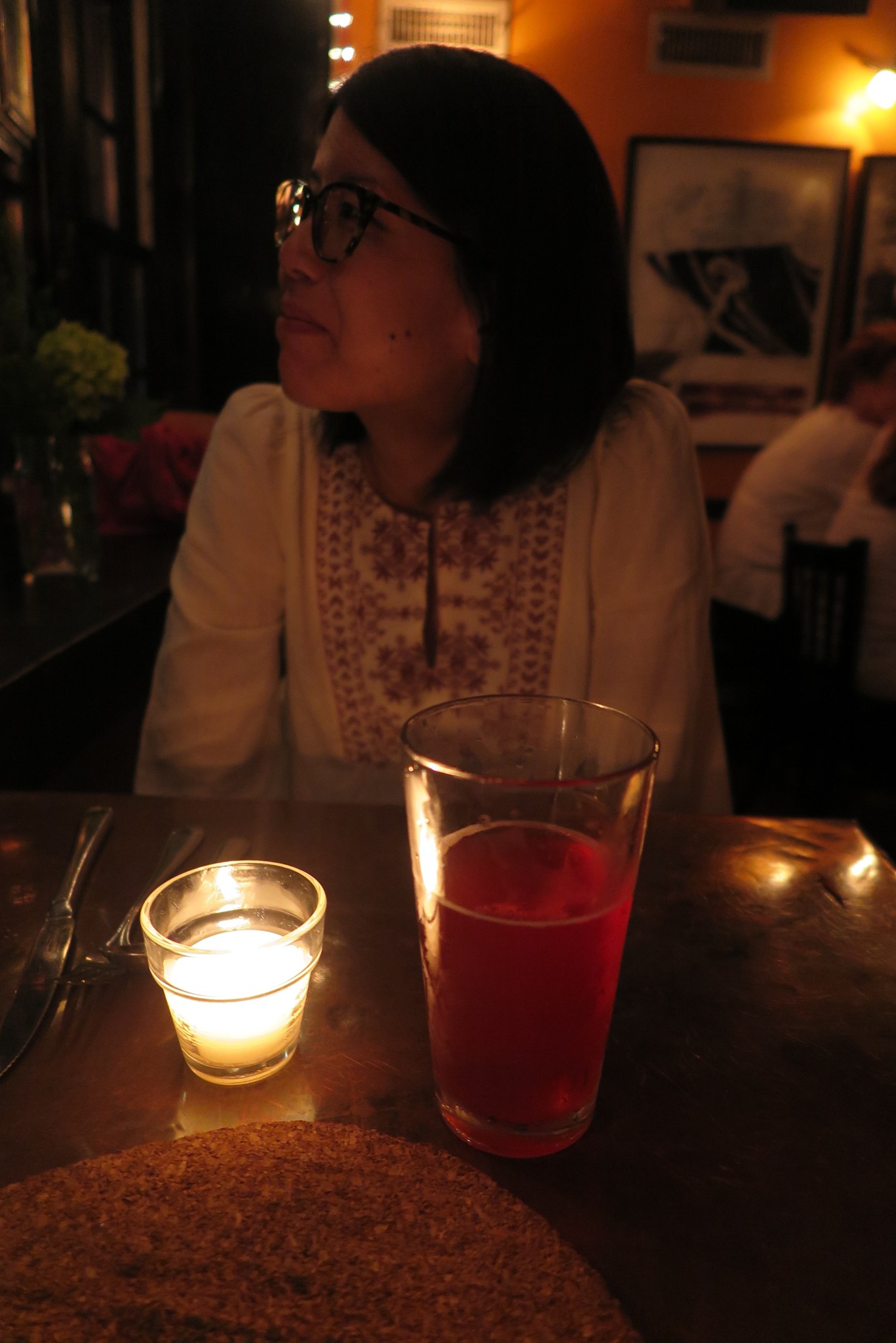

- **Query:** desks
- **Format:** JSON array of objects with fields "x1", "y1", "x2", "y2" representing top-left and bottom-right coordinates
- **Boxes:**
[
  {"x1": 0, "y1": 529, "x2": 183, "y2": 785},
  {"x1": 0, "y1": 776, "x2": 896, "y2": 1343}
]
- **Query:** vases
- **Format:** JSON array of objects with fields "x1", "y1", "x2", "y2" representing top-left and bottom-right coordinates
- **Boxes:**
[{"x1": 36, "y1": 416, "x2": 101, "y2": 587}]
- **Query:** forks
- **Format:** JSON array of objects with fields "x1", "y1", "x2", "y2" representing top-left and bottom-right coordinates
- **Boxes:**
[{"x1": 51, "y1": 824, "x2": 205, "y2": 988}]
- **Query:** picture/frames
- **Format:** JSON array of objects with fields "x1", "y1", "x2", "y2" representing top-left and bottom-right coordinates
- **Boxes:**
[
  {"x1": 846, "y1": 154, "x2": 896, "y2": 338},
  {"x1": 626, "y1": 135, "x2": 851, "y2": 455}
]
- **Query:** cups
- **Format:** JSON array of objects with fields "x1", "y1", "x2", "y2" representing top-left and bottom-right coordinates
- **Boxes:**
[
  {"x1": 140, "y1": 859, "x2": 326, "y2": 1087},
  {"x1": 401, "y1": 693, "x2": 662, "y2": 1160}
]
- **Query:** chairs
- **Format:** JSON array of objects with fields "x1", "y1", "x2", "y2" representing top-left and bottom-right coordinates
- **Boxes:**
[{"x1": 694, "y1": 499, "x2": 895, "y2": 859}]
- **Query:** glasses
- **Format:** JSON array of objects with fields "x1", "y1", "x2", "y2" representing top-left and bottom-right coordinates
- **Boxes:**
[{"x1": 272, "y1": 177, "x2": 455, "y2": 270}]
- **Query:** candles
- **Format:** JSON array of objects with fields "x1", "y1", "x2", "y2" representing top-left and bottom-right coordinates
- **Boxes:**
[{"x1": 164, "y1": 926, "x2": 315, "y2": 1066}]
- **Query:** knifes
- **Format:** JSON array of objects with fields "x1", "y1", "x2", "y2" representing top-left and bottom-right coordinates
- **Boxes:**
[{"x1": 0, "y1": 804, "x2": 115, "y2": 1079}]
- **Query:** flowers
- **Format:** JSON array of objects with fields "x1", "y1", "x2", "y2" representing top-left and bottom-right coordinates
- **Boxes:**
[{"x1": 35, "y1": 321, "x2": 130, "y2": 432}]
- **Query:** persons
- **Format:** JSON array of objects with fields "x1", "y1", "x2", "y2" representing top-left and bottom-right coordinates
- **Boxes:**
[
  {"x1": 130, "y1": 41, "x2": 749, "y2": 818},
  {"x1": 709, "y1": 318, "x2": 896, "y2": 819}
]
